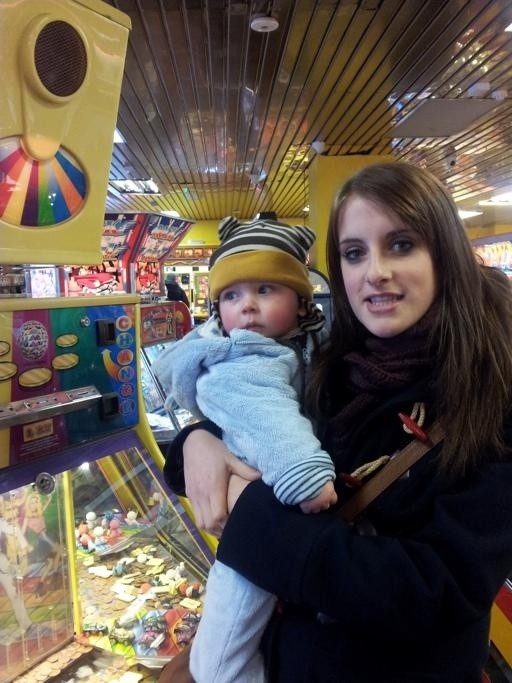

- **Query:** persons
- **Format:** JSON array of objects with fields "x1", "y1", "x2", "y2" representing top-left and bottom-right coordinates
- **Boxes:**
[
  {"x1": 162, "y1": 159, "x2": 511, "y2": 682},
  {"x1": 164, "y1": 277, "x2": 192, "y2": 308},
  {"x1": 151, "y1": 213, "x2": 341, "y2": 682}
]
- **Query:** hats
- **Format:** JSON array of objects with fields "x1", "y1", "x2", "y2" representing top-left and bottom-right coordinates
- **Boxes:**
[{"x1": 206, "y1": 214, "x2": 318, "y2": 304}]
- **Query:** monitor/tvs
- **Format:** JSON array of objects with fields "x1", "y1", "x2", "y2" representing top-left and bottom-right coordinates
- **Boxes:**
[
  {"x1": 25, "y1": 265, "x2": 60, "y2": 300},
  {"x1": 138, "y1": 350, "x2": 164, "y2": 418},
  {"x1": 145, "y1": 338, "x2": 180, "y2": 369}
]
